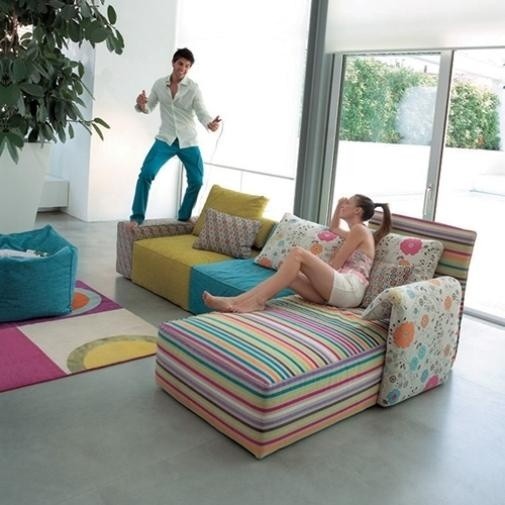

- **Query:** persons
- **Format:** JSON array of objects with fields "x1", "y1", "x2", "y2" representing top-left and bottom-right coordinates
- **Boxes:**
[
  {"x1": 202, "y1": 194, "x2": 392, "y2": 313},
  {"x1": 130, "y1": 48, "x2": 222, "y2": 227}
]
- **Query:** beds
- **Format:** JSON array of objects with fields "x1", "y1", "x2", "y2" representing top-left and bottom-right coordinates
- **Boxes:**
[{"x1": 155, "y1": 216, "x2": 478, "y2": 459}]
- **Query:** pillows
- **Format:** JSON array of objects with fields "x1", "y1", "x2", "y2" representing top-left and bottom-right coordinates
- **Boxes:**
[
  {"x1": 193, "y1": 208, "x2": 262, "y2": 260},
  {"x1": 251, "y1": 212, "x2": 443, "y2": 308},
  {"x1": 192, "y1": 185, "x2": 268, "y2": 235}
]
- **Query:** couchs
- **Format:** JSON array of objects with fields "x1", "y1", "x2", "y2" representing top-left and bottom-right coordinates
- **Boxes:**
[{"x1": 115, "y1": 209, "x2": 298, "y2": 313}]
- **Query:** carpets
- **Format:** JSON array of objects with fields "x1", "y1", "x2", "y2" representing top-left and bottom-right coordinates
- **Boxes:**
[{"x1": 0, "y1": 279, "x2": 158, "y2": 393}]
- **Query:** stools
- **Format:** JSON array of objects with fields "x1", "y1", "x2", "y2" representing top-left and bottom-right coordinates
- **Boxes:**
[{"x1": 0, "y1": 224, "x2": 79, "y2": 322}]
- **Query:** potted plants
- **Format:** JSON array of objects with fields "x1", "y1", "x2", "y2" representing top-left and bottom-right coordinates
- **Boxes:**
[{"x1": 1, "y1": 0, "x2": 123, "y2": 233}]
